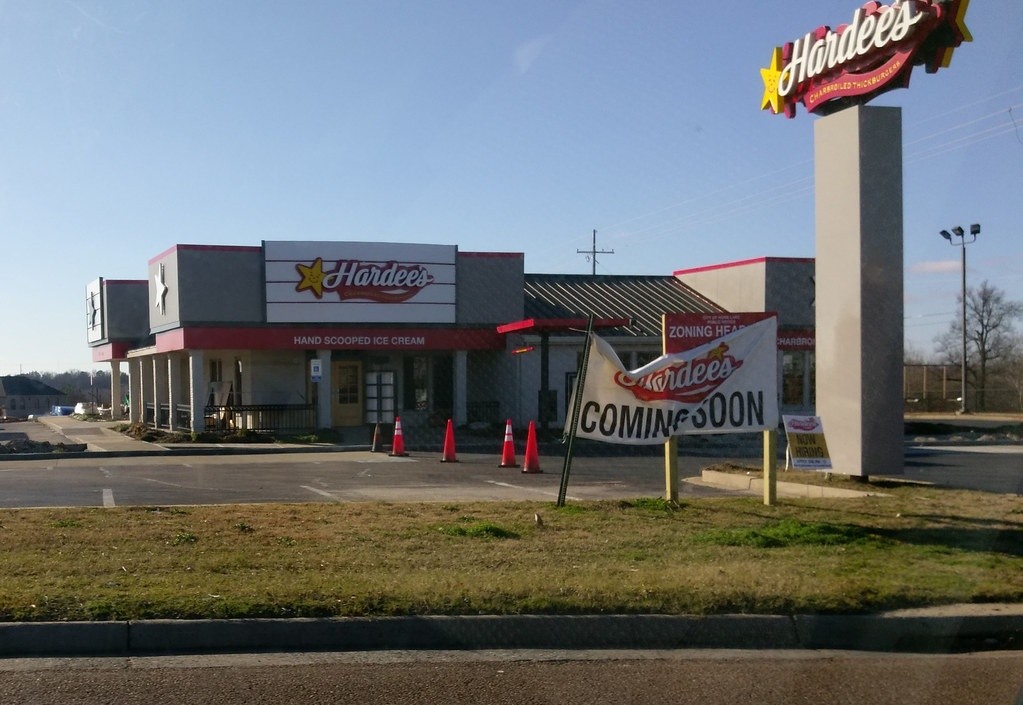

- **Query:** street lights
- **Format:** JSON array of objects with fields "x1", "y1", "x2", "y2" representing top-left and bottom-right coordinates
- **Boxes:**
[{"x1": 938, "y1": 222, "x2": 983, "y2": 414}]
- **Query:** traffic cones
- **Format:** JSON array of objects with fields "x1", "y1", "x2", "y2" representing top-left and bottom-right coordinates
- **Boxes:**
[
  {"x1": 440, "y1": 419, "x2": 460, "y2": 462},
  {"x1": 371, "y1": 422, "x2": 386, "y2": 452},
  {"x1": 520, "y1": 419, "x2": 544, "y2": 474},
  {"x1": 387, "y1": 417, "x2": 410, "y2": 457},
  {"x1": 498, "y1": 419, "x2": 522, "y2": 467}
]
